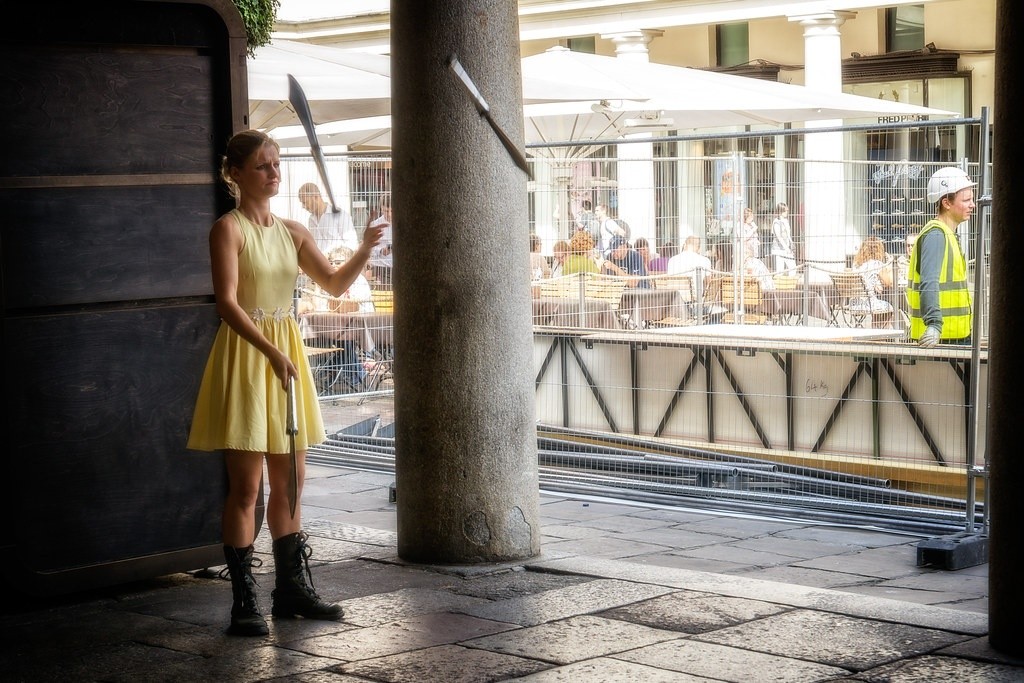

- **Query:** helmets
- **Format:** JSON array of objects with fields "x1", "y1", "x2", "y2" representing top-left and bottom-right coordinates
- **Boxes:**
[{"x1": 927, "y1": 167, "x2": 978, "y2": 203}]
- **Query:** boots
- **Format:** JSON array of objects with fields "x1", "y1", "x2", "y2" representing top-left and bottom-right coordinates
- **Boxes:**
[
  {"x1": 271, "y1": 530, "x2": 344, "y2": 620},
  {"x1": 219, "y1": 544, "x2": 269, "y2": 635}
]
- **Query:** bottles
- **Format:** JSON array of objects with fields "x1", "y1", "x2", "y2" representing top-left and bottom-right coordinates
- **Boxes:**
[{"x1": 389, "y1": 482, "x2": 397, "y2": 503}]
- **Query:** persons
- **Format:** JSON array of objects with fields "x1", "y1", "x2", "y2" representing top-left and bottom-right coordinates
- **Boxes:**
[
  {"x1": 908, "y1": 165, "x2": 980, "y2": 347},
  {"x1": 531, "y1": 202, "x2": 917, "y2": 329},
  {"x1": 187, "y1": 130, "x2": 388, "y2": 637},
  {"x1": 296, "y1": 183, "x2": 392, "y2": 395}
]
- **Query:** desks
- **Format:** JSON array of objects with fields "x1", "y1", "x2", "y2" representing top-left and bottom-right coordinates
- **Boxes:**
[
  {"x1": 304, "y1": 311, "x2": 394, "y2": 405},
  {"x1": 715, "y1": 290, "x2": 830, "y2": 326},
  {"x1": 872, "y1": 284, "x2": 914, "y2": 343},
  {"x1": 797, "y1": 283, "x2": 847, "y2": 328},
  {"x1": 615, "y1": 288, "x2": 688, "y2": 330},
  {"x1": 532, "y1": 297, "x2": 622, "y2": 329}
]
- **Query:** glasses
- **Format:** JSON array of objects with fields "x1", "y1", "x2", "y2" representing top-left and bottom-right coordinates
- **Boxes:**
[{"x1": 329, "y1": 260, "x2": 347, "y2": 265}]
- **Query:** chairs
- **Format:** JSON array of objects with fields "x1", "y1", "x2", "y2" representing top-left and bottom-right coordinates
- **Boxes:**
[
  {"x1": 719, "y1": 276, "x2": 768, "y2": 325},
  {"x1": 773, "y1": 275, "x2": 798, "y2": 289},
  {"x1": 364, "y1": 290, "x2": 394, "y2": 390},
  {"x1": 829, "y1": 274, "x2": 894, "y2": 328},
  {"x1": 649, "y1": 276, "x2": 706, "y2": 328},
  {"x1": 540, "y1": 278, "x2": 572, "y2": 298},
  {"x1": 586, "y1": 278, "x2": 627, "y2": 316},
  {"x1": 692, "y1": 273, "x2": 722, "y2": 325},
  {"x1": 304, "y1": 346, "x2": 345, "y2": 396}
]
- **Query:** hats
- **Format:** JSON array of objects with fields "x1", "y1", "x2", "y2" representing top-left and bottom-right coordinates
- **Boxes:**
[{"x1": 603, "y1": 235, "x2": 626, "y2": 253}]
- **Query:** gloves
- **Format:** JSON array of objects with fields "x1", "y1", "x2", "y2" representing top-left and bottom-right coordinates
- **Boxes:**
[{"x1": 918, "y1": 326, "x2": 940, "y2": 349}]
